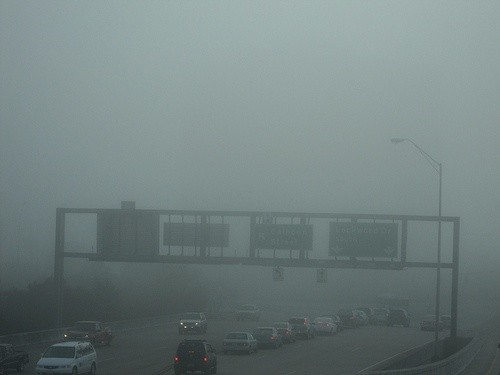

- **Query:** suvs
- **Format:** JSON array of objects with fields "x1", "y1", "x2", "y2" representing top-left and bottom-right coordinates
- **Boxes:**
[
  {"x1": 177, "y1": 312, "x2": 208, "y2": 335},
  {"x1": 35, "y1": 341, "x2": 98, "y2": 375},
  {"x1": 287, "y1": 317, "x2": 316, "y2": 341},
  {"x1": 174, "y1": 338, "x2": 218, "y2": 375},
  {"x1": 387, "y1": 308, "x2": 411, "y2": 328}
]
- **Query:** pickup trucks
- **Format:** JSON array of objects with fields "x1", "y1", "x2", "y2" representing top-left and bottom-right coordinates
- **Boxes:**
[
  {"x1": 0, "y1": 344, "x2": 30, "y2": 373},
  {"x1": 63, "y1": 320, "x2": 112, "y2": 349}
]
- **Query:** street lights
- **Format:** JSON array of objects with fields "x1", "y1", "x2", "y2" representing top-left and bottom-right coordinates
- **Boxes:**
[{"x1": 390, "y1": 137, "x2": 442, "y2": 361}]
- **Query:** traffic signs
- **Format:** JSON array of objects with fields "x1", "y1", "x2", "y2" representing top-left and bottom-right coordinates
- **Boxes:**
[{"x1": 253, "y1": 223, "x2": 313, "y2": 250}]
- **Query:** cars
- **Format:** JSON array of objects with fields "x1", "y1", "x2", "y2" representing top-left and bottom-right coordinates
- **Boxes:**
[
  {"x1": 327, "y1": 306, "x2": 391, "y2": 332},
  {"x1": 272, "y1": 322, "x2": 297, "y2": 344},
  {"x1": 439, "y1": 314, "x2": 451, "y2": 329},
  {"x1": 419, "y1": 315, "x2": 437, "y2": 332},
  {"x1": 253, "y1": 327, "x2": 283, "y2": 349},
  {"x1": 232, "y1": 304, "x2": 261, "y2": 322},
  {"x1": 311, "y1": 317, "x2": 338, "y2": 336},
  {"x1": 221, "y1": 331, "x2": 259, "y2": 355}
]
